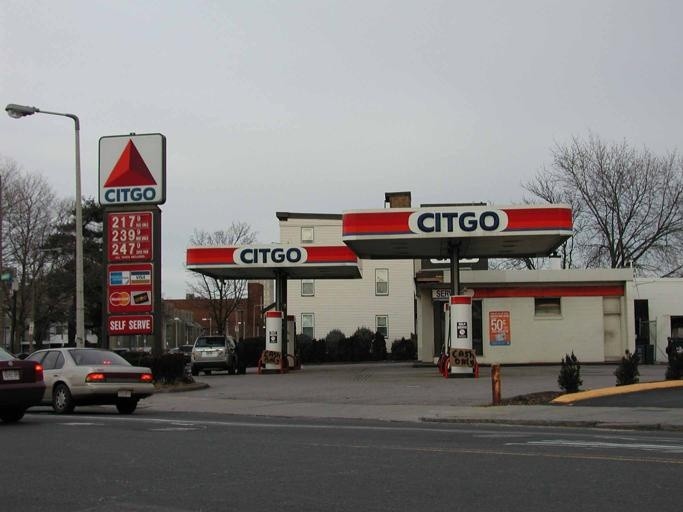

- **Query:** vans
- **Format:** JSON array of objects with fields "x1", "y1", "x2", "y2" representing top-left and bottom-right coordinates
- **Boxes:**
[{"x1": 191, "y1": 336, "x2": 238, "y2": 375}]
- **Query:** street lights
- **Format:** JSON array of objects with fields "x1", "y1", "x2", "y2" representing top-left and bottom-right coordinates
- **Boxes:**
[
  {"x1": 238, "y1": 321, "x2": 245, "y2": 340},
  {"x1": 5, "y1": 104, "x2": 86, "y2": 348},
  {"x1": 202, "y1": 317, "x2": 211, "y2": 336},
  {"x1": 174, "y1": 317, "x2": 180, "y2": 347}
]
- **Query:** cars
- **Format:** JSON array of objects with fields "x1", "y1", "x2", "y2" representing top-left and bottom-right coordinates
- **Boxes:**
[
  {"x1": 0, "y1": 346, "x2": 156, "y2": 421},
  {"x1": 168, "y1": 345, "x2": 193, "y2": 365}
]
